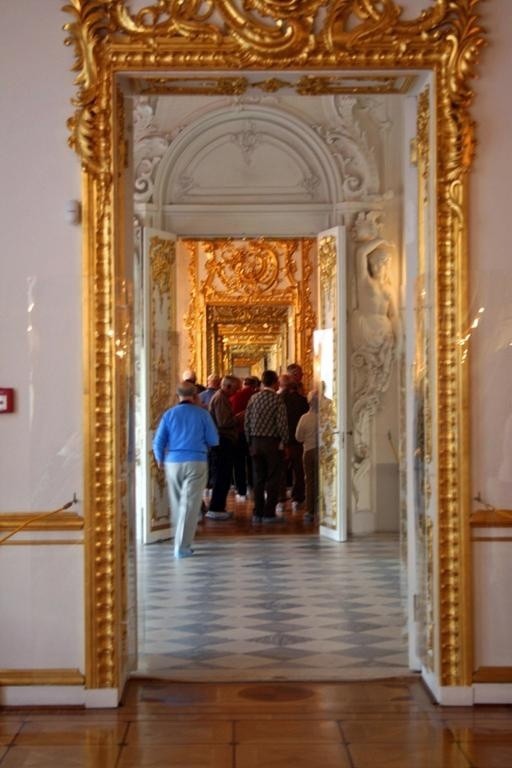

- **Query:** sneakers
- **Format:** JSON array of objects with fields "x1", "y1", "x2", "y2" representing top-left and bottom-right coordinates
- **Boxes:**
[
  {"x1": 175, "y1": 546, "x2": 193, "y2": 557},
  {"x1": 203, "y1": 483, "x2": 314, "y2": 523}
]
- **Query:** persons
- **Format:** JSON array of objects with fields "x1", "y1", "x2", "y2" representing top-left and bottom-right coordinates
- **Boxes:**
[
  {"x1": 180, "y1": 363, "x2": 319, "y2": 526},
  {"x1": 151, "y1": 381, "x2": 220, "y2": 559}
]
